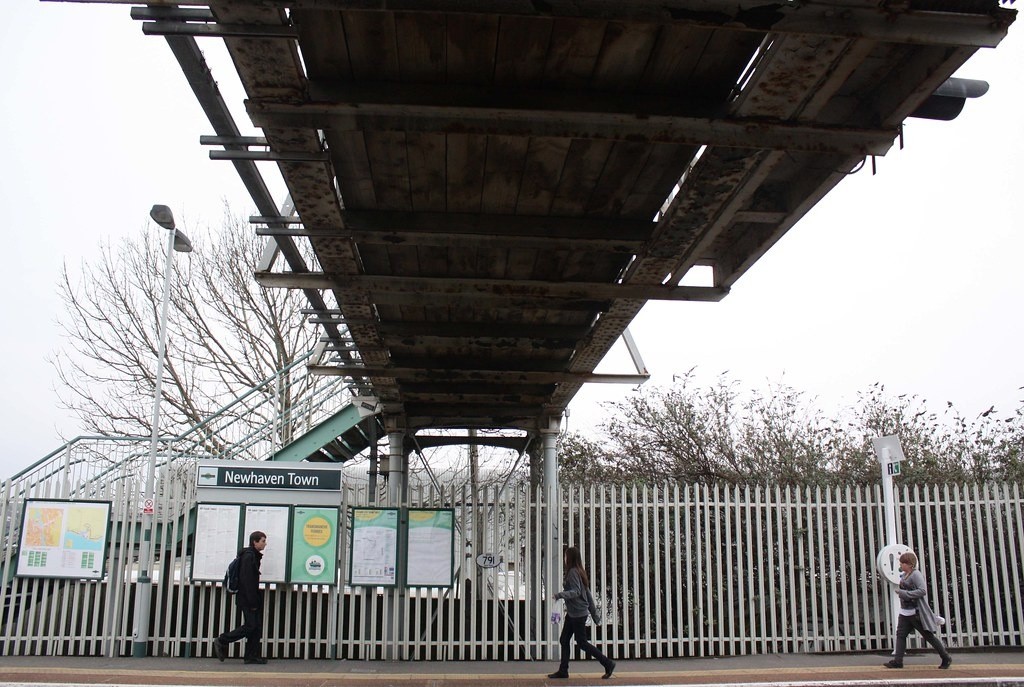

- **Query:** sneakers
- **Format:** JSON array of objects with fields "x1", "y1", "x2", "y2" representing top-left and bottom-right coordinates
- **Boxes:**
[
  {"x1": 883, "y1": 659, "x2": 903, "y2": 668},
  {"x1": 938, "y1": 655, "x2": 952, "y2": 669},
  {"x1": 601, "y1": 661, "x2": 616, "y2": 679},
  {"x1": 547, "y1": 670, "x2": 569, "y2": 678}
]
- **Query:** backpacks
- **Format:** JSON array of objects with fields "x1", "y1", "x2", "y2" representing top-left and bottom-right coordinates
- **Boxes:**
[{"x1": 223, "y1": 547, "x2": 254, "y2": 595}]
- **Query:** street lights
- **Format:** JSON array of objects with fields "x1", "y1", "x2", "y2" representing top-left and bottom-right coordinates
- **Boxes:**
[{"x1": 129, "y1": 203, "x2": 195, "y2": 659}]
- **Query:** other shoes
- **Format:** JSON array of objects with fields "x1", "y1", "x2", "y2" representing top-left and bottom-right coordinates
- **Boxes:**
[
  {"x1": 214, "y1": 638, "x2": 225, "y2": 662},
  {"x1": 244, "y1": 656, "x2": 268, "y2": 664}
]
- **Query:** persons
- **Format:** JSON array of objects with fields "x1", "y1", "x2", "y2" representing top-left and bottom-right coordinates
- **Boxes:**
[
  {"x1": 883, "y1": 553, "x2": 953, "y2": 669},
  {"x1": 215, "y1": 531, "x2": 267, "y2": 664},
  {"x1": 547, "y1": 546, "x2": 616, "y2": 679}
]
